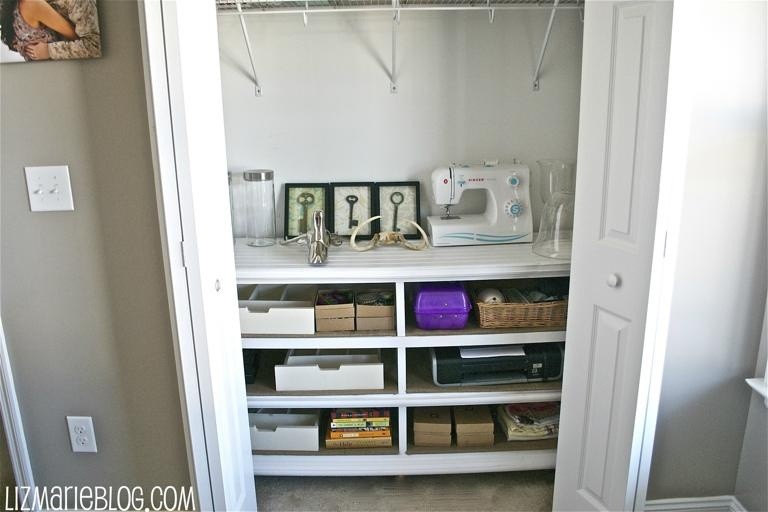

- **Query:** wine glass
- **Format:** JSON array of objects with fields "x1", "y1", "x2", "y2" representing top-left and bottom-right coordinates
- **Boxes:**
[{"x1": 534, "y1": 157, "x2": 576, "y2": 244}]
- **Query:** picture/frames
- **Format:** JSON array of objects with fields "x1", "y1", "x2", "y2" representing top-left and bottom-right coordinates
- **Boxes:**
[{"x1": 283, "y1": 181, "x2": 421, "y2": 243}]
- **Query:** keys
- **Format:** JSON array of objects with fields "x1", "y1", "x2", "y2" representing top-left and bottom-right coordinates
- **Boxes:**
[
  {"x1": 390, "y1": 192, "x2": 404, "y2": 233},
  {"x1": 346, "y1": 195, "x2": 360, "y2": 229},
  {"x1": 296, "y1": 192, "x2": 315, "y2": 234}
]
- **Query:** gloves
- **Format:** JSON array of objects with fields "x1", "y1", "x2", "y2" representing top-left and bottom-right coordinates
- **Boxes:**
[{"x1": 471, "y1": 283, "x2": 567, "y2": 328}]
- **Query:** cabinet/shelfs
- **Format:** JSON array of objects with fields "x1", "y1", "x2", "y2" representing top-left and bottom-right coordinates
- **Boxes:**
[{"x1": 234, "y1": 233, "x2": 570, "y2": 476}]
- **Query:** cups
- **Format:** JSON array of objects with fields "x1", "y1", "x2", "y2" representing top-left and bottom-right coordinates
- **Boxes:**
[
  {"x1": 534, "y1": 192, "x2": 572, "y2": 261},
  {"x1": 243, "y1": 166, "x2": 277, "y2": 251}
]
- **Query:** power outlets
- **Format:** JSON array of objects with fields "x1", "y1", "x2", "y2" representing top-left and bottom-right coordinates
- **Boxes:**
[{"x1": 67, "y1": 416, "x2": 98, "y2": 452}]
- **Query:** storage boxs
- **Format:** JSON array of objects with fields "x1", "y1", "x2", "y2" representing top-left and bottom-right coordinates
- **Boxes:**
[
  {"x1": 415, "y1": 282, "x2": 473, "y2": 331},
  {"x1": 414, "y1": 407, "x2": 452, "y2": 446},
  {"x1": 453, "y1": 404, "x2": 495, "y2": 445}
]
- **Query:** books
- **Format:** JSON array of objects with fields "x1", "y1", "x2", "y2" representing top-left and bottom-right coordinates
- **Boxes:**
[{"x1": 322, "y1": 405, "x2": 394, "y2": 449}]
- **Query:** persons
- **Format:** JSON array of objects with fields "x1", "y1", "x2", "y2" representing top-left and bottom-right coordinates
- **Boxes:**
[
  {"x1": 25, "y1": 0, "x2": 104, "y2": 60},
  {"x1": 0, "y1": 1, "x2": 70, "y2": 60}
]
